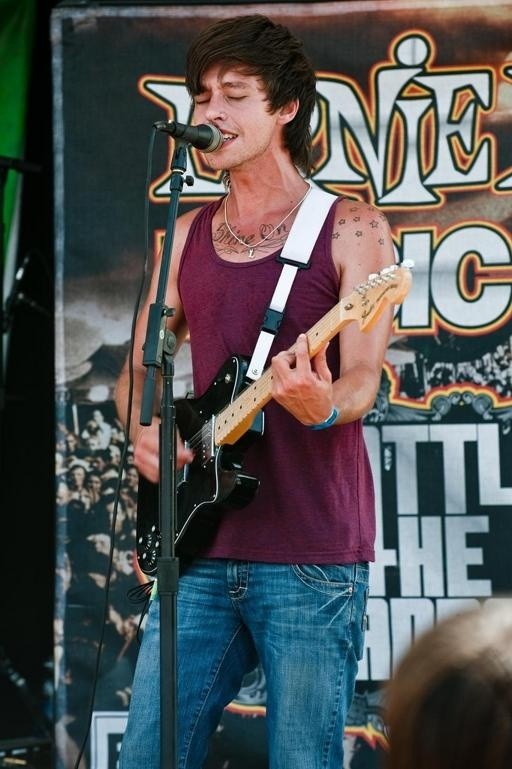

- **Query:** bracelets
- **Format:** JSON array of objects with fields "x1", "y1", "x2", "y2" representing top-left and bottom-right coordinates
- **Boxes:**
[{"x1": 306, "y1": 407, "x2": 340, "y2": 431}]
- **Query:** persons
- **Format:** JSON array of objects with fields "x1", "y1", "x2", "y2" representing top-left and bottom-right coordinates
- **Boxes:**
[
  {"x1": 45, "y1": 407, "x2": 157, "y2": 724},
  {"x1": 383, "y1": 600, "x2": 512, "y2": 769},
  {"x1": 114, "y1": 15, "x2": 396, "y2": 769}
]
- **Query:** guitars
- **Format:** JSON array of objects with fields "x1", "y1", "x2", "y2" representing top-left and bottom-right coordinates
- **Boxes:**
[{"x1": 136, "y1": 259, "x2": 414, "y2": 577}]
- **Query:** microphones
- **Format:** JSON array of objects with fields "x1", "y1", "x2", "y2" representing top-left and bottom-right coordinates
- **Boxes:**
[{"x1": 154, "y1": 120, "x2": 224, "y2": 153}]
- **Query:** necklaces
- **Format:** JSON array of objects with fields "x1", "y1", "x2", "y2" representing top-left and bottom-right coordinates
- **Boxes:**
[{"x1": 224, "y1": 179, "x2": 312, "y2": 257}]
